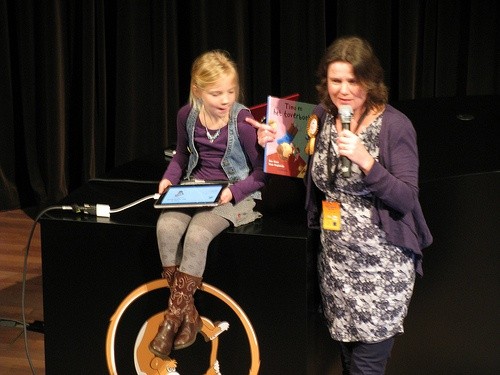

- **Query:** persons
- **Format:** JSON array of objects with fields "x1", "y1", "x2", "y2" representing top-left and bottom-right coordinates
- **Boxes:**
[
  {"x1": 245, "y1": 36, "x2": 434, "y2": 374},
  {"x1": 148, "y1": 50, "x2": 273, "y2": 359}
]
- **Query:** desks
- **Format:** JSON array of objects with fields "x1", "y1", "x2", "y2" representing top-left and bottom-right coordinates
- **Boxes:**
[{"x1": 39, "y1": 181, "x2": 344, "y2": 375}]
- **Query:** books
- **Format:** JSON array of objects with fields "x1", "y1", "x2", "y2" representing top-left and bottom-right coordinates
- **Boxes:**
[{"x1": 264, "y1": 95, "x2": 319, "y2": 179}]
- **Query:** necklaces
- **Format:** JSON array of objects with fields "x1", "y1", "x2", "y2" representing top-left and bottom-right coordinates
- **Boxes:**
[{"x1": 203, "y1": 111, "x2": 225, "y2": 144}]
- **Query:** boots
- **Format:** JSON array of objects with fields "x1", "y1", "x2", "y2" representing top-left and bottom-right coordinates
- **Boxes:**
[{"x1": 147, "y1": 266, "x2": 203, "y2": 357}]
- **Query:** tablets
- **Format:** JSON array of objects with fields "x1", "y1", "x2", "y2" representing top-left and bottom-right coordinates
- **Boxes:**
[{"x1": 154, "y1": 182, "x2": 229, "y2": 208}]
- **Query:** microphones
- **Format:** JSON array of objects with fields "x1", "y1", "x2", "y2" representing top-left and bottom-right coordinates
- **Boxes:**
[{"x1": 338, "y1": 105, "x2": 353, "y2": 177}]
